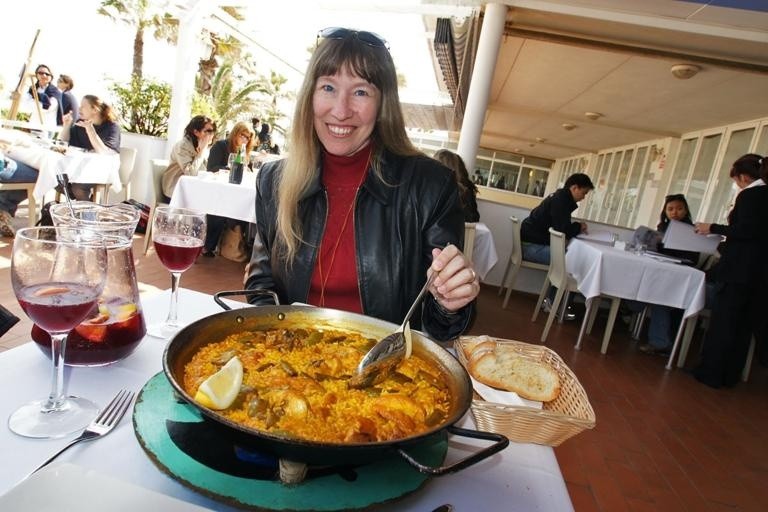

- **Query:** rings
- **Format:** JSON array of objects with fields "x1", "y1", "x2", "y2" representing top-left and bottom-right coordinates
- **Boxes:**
[{"x1": 468, "y1": 267, "x2": 475, "y2": 283}]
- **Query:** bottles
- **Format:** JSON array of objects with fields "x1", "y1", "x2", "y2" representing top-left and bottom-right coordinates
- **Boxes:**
[{"x1": 228, "y1": 144, "x2": 247, "y2": 183}]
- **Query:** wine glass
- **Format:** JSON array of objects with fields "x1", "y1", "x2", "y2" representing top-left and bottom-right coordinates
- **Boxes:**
[
  {"x1": 151, "y1": 206, "x2": 206, "y2": 331},
  {"x1": 7, "y1": 221, "x2": 105, "y2": 439}
]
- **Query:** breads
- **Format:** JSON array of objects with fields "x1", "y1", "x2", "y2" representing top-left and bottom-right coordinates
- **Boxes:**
[{"x1": 465, "y1": 334, "x2": 561, "y2": 402}]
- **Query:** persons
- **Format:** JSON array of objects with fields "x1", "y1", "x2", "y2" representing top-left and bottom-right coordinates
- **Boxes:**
[
  {"x1": 519, "y1": 170, "x2": 593, "y2": 331},
  {"x1": 206, "y1": 118, "x2": 272, "y2": 172},
  {"x1": 686, "y1": 153, "x2": 766, "y2": 387},
  {"x1": 430, "y1": 144, "x2": 481, "y2": 225},
  {"x1": 269, "y1": 144, "x2": 281, "y2": 154},
  {"x1": 245, "y1": 28, "x2": 486, "y2": 342},
  {"x1": 29, "y1": 64, "x2": 121, "y2": 201},
  {"x1": 630, "y1": 191, "x2": 694, "y2": 356},
  {"x1": 161, "y1": 115, "x2": 228, "y2": 257},
  {"x1": 466, "y1": 166, "x2": 543, "y2": 197}
]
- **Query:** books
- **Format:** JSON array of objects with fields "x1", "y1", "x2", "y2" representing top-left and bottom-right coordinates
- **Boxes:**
[{"x1": 643, "y1": 251, "x2": 693, "y2": 266}]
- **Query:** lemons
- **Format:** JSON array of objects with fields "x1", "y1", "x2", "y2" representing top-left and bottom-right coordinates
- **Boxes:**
[{"x1": 194, "y1": 355, "x2": 243, "y2": 410}]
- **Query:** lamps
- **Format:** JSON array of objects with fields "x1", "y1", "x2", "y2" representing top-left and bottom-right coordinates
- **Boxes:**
[
  {"x1": 513, "y1": 133, "x2": 546, "y2": 155},
  {"x1": 671, "y1": 64, "x2": 701, "y2": 80},
  {"x1": 584, "y1": 110, "x2": 605, "y2": 121},
  {"x1": 562, "y1": 123, "x2": 576, "y2": 131}
]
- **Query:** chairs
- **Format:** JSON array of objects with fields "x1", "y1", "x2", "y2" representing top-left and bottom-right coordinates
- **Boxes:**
[
  {"x1": 457, "y1": 221, "x2": 478, "y2": 261},
  {"x1": 530, "y1": 227, "x2": 622, "y2": 356},
  {"x1": 497, "y1": 216, "x2": 553, "y2": 308},
  {"x1": 666, "y1": 254, "x2": 763, "y2": 381}
]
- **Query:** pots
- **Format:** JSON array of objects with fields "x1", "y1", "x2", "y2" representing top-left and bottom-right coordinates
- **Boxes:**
[{"x1": 164, "y1": 288, "x2": 510, "y2": 477}]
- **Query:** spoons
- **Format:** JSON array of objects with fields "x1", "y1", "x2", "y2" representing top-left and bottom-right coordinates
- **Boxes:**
[{"x1": 356, "y1": 268, "x2": 437, "y2": 385}]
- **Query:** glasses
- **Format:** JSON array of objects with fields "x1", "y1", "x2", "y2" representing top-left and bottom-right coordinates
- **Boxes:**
[
  {"x1": 317, "y1": 27, "x2": 390, "y2": 50},
  {"x1": 35, "y1": 72, "x2": 51, "y2": 76},
  {"x1": 665, "y1": 194, "x2": 684, "y2": 202}
]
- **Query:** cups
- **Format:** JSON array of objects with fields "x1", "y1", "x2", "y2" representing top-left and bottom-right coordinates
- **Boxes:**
[
  {"x1": 633, "y1": 241, "x2": 648, "y2": 256},
  {"x1": 30, "y1": 129, "x2": 81, "y2": 153}
]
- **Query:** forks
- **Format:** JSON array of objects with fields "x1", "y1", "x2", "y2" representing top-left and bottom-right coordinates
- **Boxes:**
[{"x1": 0, "y1": 388, "x2": 134, "y2": 497}]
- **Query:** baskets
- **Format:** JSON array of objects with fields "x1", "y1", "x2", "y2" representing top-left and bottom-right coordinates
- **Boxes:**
[{"x1": 453, "y1": 335, "x2": 596, "y2": 448}]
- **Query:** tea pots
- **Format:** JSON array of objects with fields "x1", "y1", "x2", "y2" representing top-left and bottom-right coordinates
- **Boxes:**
[{"x1": 30, "y1": 198, "x2": 149, "y2": 367}]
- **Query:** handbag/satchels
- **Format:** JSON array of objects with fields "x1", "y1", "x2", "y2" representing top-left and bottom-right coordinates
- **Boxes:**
[{"x1": 216, "y1": 219, "x2": 248, "y2": 263}]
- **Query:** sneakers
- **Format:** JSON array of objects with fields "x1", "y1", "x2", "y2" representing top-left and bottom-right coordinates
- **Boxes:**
[
  {"x1": 639, "y1": 344, "x2": 672, "y2": 353},
  {"x1": 200, "y1": 251, "x2": 215, "y2": 257},
  {"x1": 0, "y1": 210, "x2": 16, "y2": 237},
  {"x1": 543, "y1": 297, "x2": 576, "y2": 321}
]
